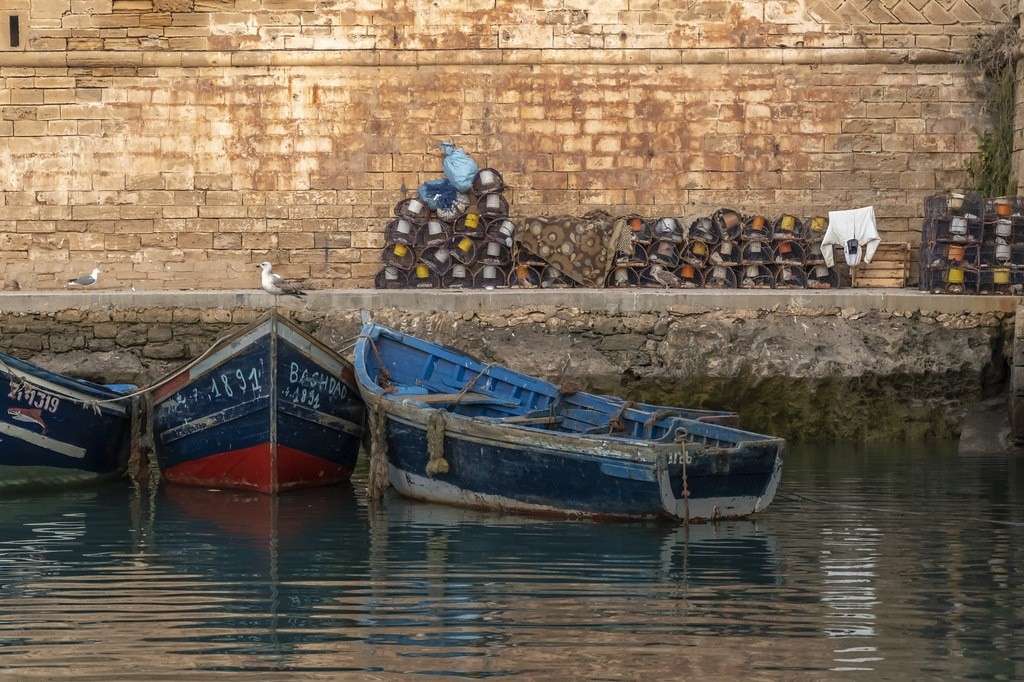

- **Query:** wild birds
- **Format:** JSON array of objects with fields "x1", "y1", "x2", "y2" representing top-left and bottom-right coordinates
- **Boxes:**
[
  {"x1": 67, "y1": 267, "x2": 102, "y2": 293},
  {"x1": 256, "y1": 259, "x2": 311, "y2": 310}
]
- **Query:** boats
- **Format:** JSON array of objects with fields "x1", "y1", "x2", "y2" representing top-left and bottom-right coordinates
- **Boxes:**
[
  {"x1": 353, "y1": 313, "x2": 788, "y2": 519},
  {"x1": 129, "y1": 304, "x2": 365, "y2": 494},
  {"x1": 0, "y1": 352, "x2": 144, "y2": 495}
]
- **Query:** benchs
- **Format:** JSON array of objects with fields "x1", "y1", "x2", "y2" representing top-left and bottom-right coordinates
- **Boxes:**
[
  {"x1": 475, "y1": 416, "x2": 563, "y2": 424},
  {"x1": 397, "y1": 394, "x2": 492, "y2": 404}
]
